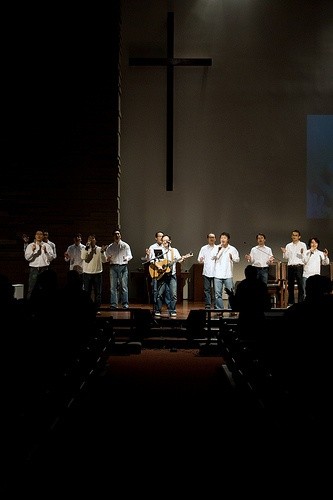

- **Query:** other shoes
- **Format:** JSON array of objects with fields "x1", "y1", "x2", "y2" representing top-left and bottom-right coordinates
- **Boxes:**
[
  {"x1": 217, "y1": 312, "x2": 223, "y2": 316},
  {"x1": 170, "y1": 312, "x2": 177, "y2": 316},
  {"x1": 155, "y1": 311, "x2": 160, "y2": 315},
  {"x1": 231, "y1": 313, "x2": 238, "y2": 316}
]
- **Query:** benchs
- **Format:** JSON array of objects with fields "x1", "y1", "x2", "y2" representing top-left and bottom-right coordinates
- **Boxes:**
[{"x1": 0, "y1": 308, "x2": 332, "y2": 500}]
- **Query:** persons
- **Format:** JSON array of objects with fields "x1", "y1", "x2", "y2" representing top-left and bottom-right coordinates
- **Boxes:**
[
  {"x1": 197, "y1": 233, "x2": 220, "y2": 309},
  {"x1": 225, "y1": 265, "x2": 271, "y2": 324},
  {"x1": 40, "y1": 231, "x2": 59, "y2": 274},
  {"x1": 281, "y1": 229, "x2": 308, "y2": 309},
  {"x1": 145, "y1": 234, "x2": 194, "y2": 317},
  {"x1": 104, "y1": 230, "x2": 134, "y2": 309},
  {"x1": 211, "y1": 232, "x2": 240, "y2": 317},
  {"x1": 246, "y1": 233, "x2": 274, "y2": 287},
  {"x1": 80, "y1": 234, "x2": 108, "y2": 315},
  {"x1": 64, "y1": 233, "x2": 85, "y2": 291},
  {"x1": 276, "y1": 275, "x2": 333, "y2": 453},
  {"x1": 25, "y1": 230, "x2": 54, "y2": 299},
  {"x1": 303, "y1": 236, "x2": 330, "y2": 302}
]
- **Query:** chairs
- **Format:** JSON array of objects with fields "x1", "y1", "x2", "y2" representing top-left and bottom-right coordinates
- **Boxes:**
[
  {"x1": 267, "y1": 261, "x2": 298, "y2": 309},
  {"x1": 320, "y1": 262, "x2": 333, "y2": 294}
]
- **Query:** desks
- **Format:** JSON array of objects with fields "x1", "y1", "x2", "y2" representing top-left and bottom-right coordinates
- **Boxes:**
[{"x1": 130, "y1": 271, "x2": 190, "y2": 304}]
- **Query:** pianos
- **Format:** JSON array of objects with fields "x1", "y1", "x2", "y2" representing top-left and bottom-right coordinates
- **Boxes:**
[
  {"x1": 189, "y1": 261, "x2": 218, "y2": 301},
  {"x1": 127, "y1": 262, "x2": 186, "y2": 306},
  {"x1": 235, "y1": 259, "x2": 332, "y2": 308}
]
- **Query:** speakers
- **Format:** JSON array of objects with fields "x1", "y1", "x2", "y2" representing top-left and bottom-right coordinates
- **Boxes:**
[
  {"x1": 186, "y1": 310, "x2": 206, "y2": 335},
  {"x1": 137, "y1": 310, "x2": 151, "y2": 336}
]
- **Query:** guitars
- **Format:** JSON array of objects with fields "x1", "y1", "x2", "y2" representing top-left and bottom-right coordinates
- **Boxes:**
[{"x1": 146, "y1": 251, "x2": 195, "y2": 280}]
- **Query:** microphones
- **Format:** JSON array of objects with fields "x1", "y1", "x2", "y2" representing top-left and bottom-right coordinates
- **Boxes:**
[
  {"x1": 167, "y1": 241, "x2": 171, "y2": 244},
  {"x1": 88, "y1": 241, "x2": 91, "y2": 247}
]
